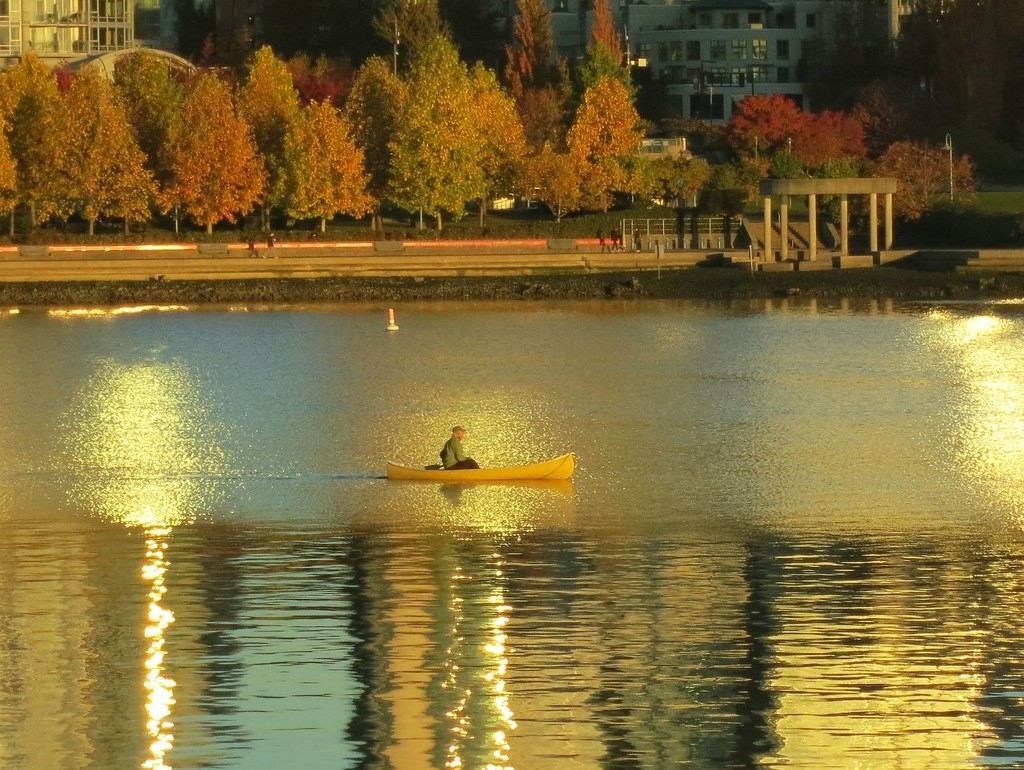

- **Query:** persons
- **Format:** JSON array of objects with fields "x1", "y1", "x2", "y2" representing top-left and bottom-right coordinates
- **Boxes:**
[
  {"x1": 1014, "y1": 213, "x2": 1024, "y2": 242},
  {"x1": 249, "y1": 240, "x2": 258, "y2": 258},
  {"x1": 600, "y1": 226, "x2": 623, "y2": 253},
  {"x1": 635, "y1": 227, "x2": 641, "y2": 252},
  {"x1": 263, "y1": 234, "x2": 278, "y2": 259},
  {"x1": 440, "y1": 426, "x2": 479, "y2": 470}
]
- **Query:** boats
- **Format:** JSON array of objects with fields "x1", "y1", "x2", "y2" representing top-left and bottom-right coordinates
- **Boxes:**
[{"x1": 386, "y1": 454, "x2": 575, "y2": 481}]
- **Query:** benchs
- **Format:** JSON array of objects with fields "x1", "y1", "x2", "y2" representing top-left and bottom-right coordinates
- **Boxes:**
[
  {"x1": 373, "y1": 241, "x2": 405, "y2": 255},
  {"x1": 196, "y1": 243, "x2": 229, "y2": 257},
  {"x1": 547, "y1": 239, "x2": 577, "y2": 253}
]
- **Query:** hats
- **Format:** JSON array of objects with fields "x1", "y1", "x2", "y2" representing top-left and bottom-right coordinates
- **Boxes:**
[{"x1": 452, "y1": 426, "x2": 467, "y2": 432}]
